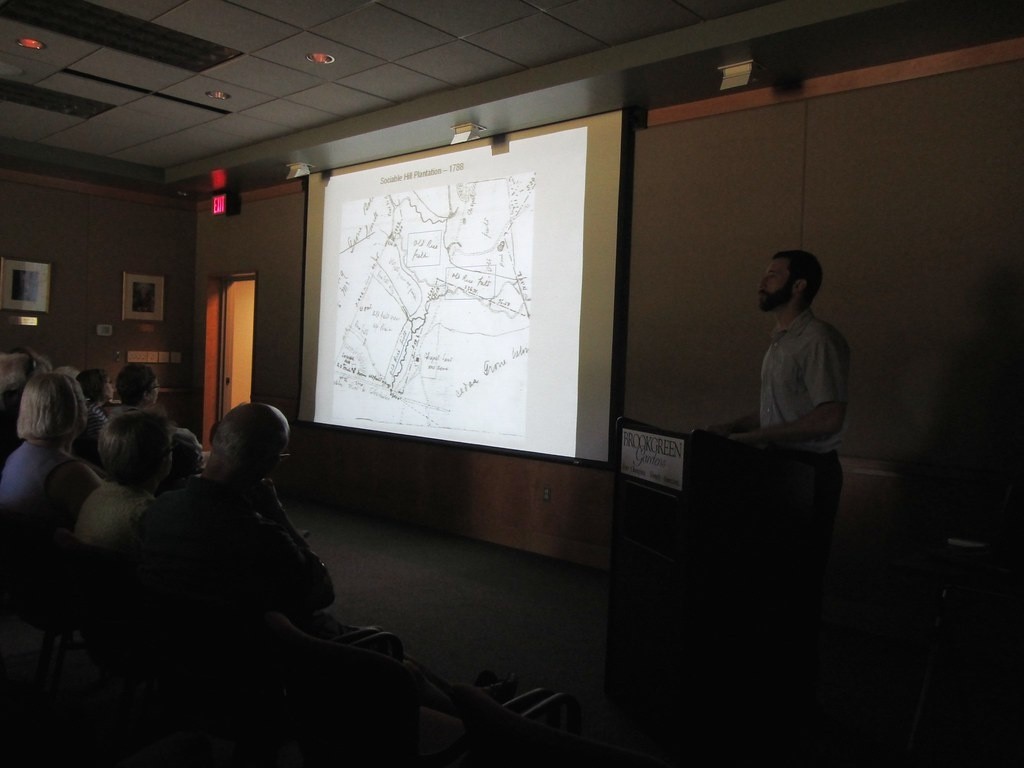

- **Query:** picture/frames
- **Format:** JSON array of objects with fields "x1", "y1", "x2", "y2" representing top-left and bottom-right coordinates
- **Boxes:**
[
  {"x1": 0, "y1": 255, "x2": 54, "y2": 315},
  {"x1": 121, "y1": 270, "x2": 164, "y2": 322}
]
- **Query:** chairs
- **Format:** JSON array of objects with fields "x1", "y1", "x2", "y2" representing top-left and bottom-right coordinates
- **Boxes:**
[
  {"x1": 900, "y1": 483, "x2": 1024, "y2": 768},
  {"x1": 0, "y1": 503, "x2": 679, "y2": 768}
]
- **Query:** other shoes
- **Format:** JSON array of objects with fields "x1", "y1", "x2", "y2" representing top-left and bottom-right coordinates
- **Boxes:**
[{"x1": 490, "y1": 673, "x2": 519, "y2": 704}]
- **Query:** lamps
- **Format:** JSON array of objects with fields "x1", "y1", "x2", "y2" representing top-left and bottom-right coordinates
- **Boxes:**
[
  {"x1": 775, "y1": 60, "x2": 802, "y2": 90},
  {"x1": 717, "y1": 59, "x2": 768, "y2": 90},
  {"x1": 286, "y1": 162, "x2": 316, "y2": 180},
  {"x1": 450, "y1": 122, "x2": 488, "y2": 146}
]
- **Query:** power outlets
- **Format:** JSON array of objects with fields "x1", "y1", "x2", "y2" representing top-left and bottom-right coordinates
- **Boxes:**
[{"x1": 147, "y1": 351, "x2": 182, "y2": 363}]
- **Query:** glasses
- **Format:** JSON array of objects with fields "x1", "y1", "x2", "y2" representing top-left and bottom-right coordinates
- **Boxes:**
[{"x1": 277, "y1": 449, "x2": 291, "y2": 460}]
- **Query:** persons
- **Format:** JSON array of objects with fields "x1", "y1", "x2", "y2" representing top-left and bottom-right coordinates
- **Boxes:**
[
  {"x1": 709, "y1": 250, "x2": 847, "y2": 717},
  {"x1": 0, "y1": 346, "x2": 517, "y2": 768}
]
